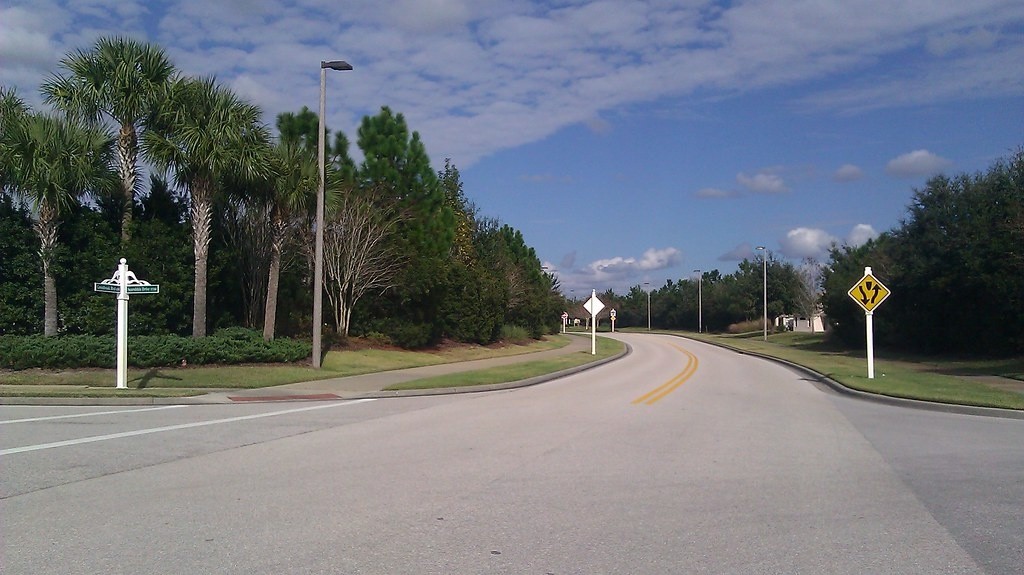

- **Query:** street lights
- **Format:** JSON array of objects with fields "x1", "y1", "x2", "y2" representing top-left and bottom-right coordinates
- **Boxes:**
[
  {"x1": 694, "y1": 270, "x2": 701, "y2": 333},
  {"x1": 755, "y1": 246, "x2": 767, "y2": 340},
  {"x1": 312, "y1": 61, "x2": 353, "y2": 370},
  {"x1": 644, "y1": 283, "x2": 650, "y2": 331}
]
